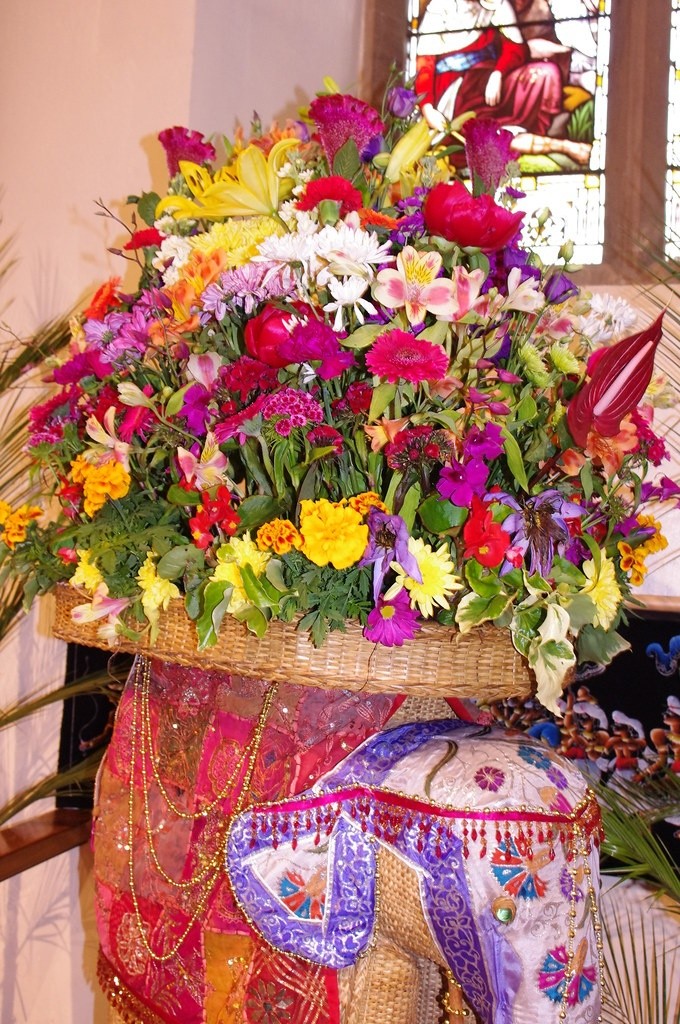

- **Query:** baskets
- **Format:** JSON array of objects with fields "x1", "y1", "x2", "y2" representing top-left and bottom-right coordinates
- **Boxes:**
[{"x1": 50, "y1": 583, "x2": 539, "y2": 1024}]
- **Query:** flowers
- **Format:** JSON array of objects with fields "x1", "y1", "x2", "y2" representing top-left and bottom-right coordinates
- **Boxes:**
[{"x1": 0, "y1": 73, "x2": 680, "y2": 720}]
